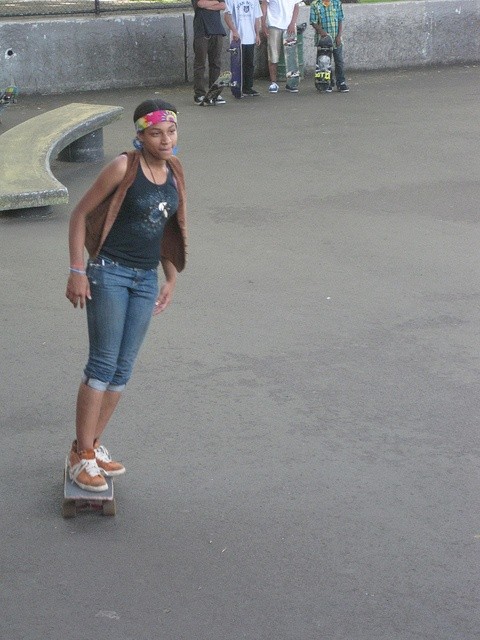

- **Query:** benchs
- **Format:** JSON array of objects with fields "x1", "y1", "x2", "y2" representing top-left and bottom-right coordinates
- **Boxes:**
[{"x1": 0, "y1": 103, "x2": 125, "y2": 216}]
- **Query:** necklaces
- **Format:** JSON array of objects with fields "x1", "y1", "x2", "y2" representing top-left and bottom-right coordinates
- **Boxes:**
[{"x1": 140, "y1": 150, "x2": 173, "y2": 218}]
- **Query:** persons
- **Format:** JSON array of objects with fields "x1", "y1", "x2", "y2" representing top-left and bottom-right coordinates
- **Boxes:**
[
  {"x1": 259, "y1": 0, "x2": 300, "y2": 94},
  {"x1": 222, "y1": 0, "x2": 263, "y2": 98},
  {"x1": 309, "y1": 0, "x2": 349, "y2": 92},
  {"x1": 64, "y1": 99, "x2": 188, "y2": 493},
  {"x1": 191, "y1": 0, "x2": 227, "y2": 105}
]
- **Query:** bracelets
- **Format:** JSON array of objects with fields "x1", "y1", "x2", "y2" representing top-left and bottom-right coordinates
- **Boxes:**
[{"x1": 70, "y1": 267, "x2": 86, "y2": 275}]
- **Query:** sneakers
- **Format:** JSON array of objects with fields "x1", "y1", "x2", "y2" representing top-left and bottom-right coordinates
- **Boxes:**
[
  {"x1": 241, "y1": 88, "x2": 260, "y2": 96},
  {"x1": 337, "y1": 81, "x2": 350, "y2": 92},
  {"x1": 285, "y1": 84, "x2": 298, "y2": 93},
  {"x1": 67, "y1": 439, "x2": 109, "y2": 494},
  {"x1": 93, "y1": 446, "x2": 126, "y2": 477},
  {"x1": 193, "y1": 96, "x2": 205, "y2": 104},
  {"x1": 326, "y1": 81, "x2": 333, "y2": 93},
  {"x1": 268, "y1": 82, "x2": 279, "y2": 94},
  {"x1": 213, "y1": 95, "x2": 226, "y2": 104}
]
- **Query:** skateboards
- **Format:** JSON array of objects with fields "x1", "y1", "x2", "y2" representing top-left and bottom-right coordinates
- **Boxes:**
[
  {"x1": 227, "y1": 38, "x2": 242, "y2": 99},
  {"x1": 62, "y1": 456, "x2": 116, "y2": 518},
  {"x1": 315, "y1": 33, "x2": 333, "y2": 92},
  {"x1": 282, "y1": 30, "x2": 301, "y2": 89},
  {"x1": 199, "y1": 71, "x2": 232, "y2": 106}
]
